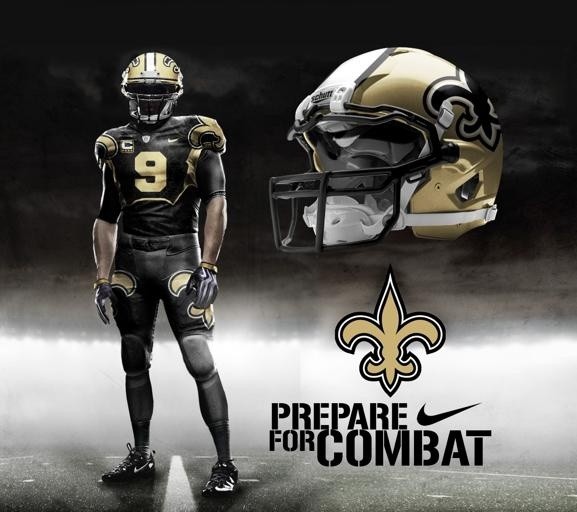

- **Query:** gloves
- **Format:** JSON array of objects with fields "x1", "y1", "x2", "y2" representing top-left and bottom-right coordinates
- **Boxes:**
[
  {"x1": 92, "y1": 278, "x2": 117, "y2": 325},
  {"x1": 186, "y1": 261, "x2": 220, "y2": 309}
]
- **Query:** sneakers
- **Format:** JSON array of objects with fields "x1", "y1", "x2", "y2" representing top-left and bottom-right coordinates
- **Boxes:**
[
  {"x1": 102, "y1": 448, "x2": 155, "y2": 483},
  {"x1": 201, "y1": 458, "x2": 239, "y2": 496}
]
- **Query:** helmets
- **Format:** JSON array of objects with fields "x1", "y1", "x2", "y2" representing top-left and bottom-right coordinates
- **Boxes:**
[
  {"x1": 290, "y1": 45, "x2": 506, "y2": 247},
  {"x1": 119, "y1": 52, "x2": 183, "y2": 117}
]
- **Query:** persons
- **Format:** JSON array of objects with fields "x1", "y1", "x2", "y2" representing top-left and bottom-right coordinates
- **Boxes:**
[{"x1": 89, "y1": 51, "x2": 240, "y2": 499}]
